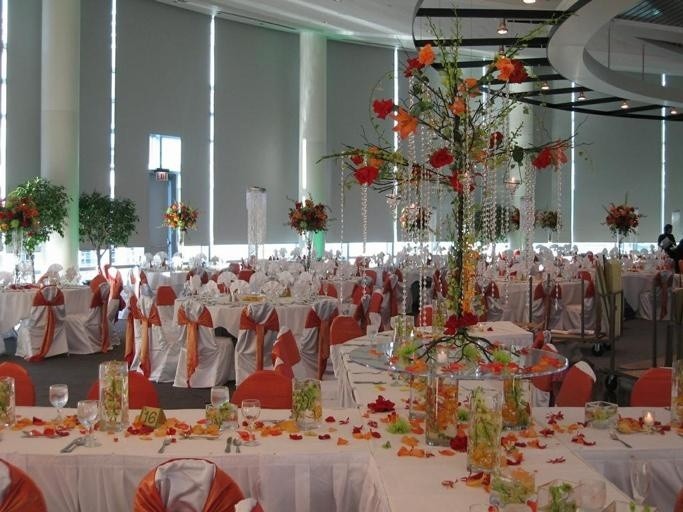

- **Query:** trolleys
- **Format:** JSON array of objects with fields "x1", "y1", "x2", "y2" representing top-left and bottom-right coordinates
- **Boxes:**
[
  {"x1": 601, "y1": 278, "x2": 683, "y2": 391},
  {"x1": 529, "y1": 274, "x2": 607, "y2": 355}
]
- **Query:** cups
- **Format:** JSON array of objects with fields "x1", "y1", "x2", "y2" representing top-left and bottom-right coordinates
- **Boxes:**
[
  {"x1": 642, "y1": 410, "x2": 655, "y2": 426},
  {"x1": 438, "y1": 348, "x2": 447, "y2": 363}
]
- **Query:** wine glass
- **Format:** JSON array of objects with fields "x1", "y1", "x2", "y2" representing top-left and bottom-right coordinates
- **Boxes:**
[
  {"x1": 50, "y1": 386, "x2": 69, "y2": 419},
  {"x1": 241, "y1": 399, "x2": 262, "y2": 443},
  {"x1": 390, "y1": 316, "x2": 395, "y2": 329},
  {"x1": 366, "y1": 326, "x2": 379, "y2": 347},
  {"x1": 577, "y1": 478, "x2": 607, "y2": 512},
  {"x1": 211, "y1": 387, "x2": 230, "y2": 437},
  {"x1": 629, "y1": 458, "x2": 651, "y2": 512},
  {"x1": 76, "y1": 401, "x2": 101, "y2": 443}
]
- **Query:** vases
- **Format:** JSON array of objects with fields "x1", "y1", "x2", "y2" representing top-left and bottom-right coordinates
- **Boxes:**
[
  {"x1": 616, "y1": 233, "x2": 625, "y2": 258},
  {"x1": 547, "y1": 232, "x2": 553, "y2": 244},
  {"x1": 304, "y1": 234, "x2": 309, "y2": 248},
  {"x1": 178, "y1": 231, "x2": 182, "y2": 247}
]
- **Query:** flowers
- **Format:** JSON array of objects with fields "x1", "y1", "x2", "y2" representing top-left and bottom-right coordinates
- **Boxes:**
[
  {"x1": 284, "y1": 190, "x2": 336, "y2": 239},
  {"x1": 318, "y1": 1, "x2": 589, "y2": 362},
  {"x1": 1, "y1": 195, "x2": 41, "y2": 243},
  {"x1": 398, "y1": 203, "x2": 434, "y2": 232},
  {"x1": 160, "y1": 199, "x2": 199, "y2": 242},
  {"x1": 602, "y1": 188, "x2": 648, "y2": 259},
  {"x1": 540, "y1": 210, "x2": 563, "y2": 240}
]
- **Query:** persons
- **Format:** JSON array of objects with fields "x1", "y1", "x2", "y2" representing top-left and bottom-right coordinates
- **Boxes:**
[
  {"x1": 658, "y1": 224, "x2": 676, "y2": 251},
  {"x1": 666, "y1": 238, "x2": 683, "y2": 273}
]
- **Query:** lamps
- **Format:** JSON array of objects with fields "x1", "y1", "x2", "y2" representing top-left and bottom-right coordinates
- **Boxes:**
[{"x1": 147, "y1": 132, "x2": 182, "y2": 183}]
